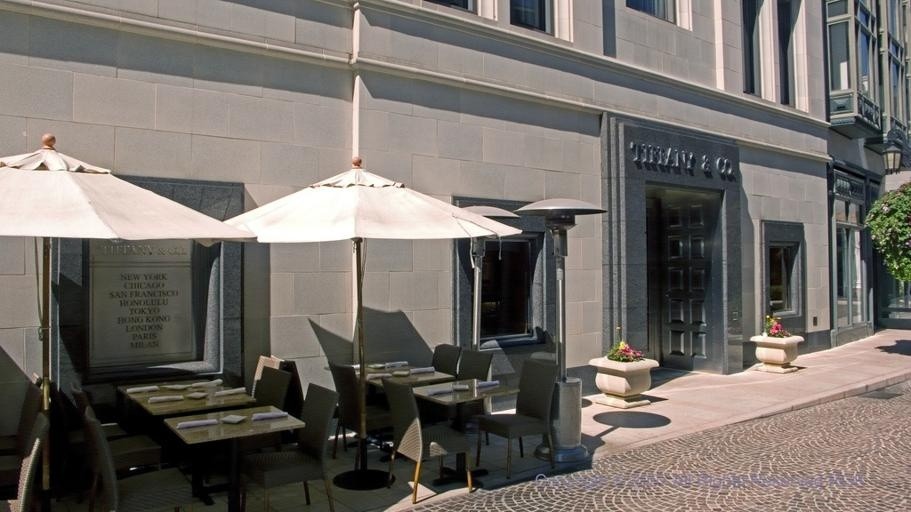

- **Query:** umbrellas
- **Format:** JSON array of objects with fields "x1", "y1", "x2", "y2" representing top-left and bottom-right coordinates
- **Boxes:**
[
  {"x1": 221, "y1": 156, "x2": 523, "y2": 485},
  {"x1": 0, "y1": 133, "x2": 257, "y2": 512}
]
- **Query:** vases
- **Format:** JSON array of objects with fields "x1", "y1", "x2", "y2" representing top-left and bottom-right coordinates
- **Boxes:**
[
  {"x1": 589, "y1": 356, "x2": 659, "y2": 409},
  {"x1": 749, "y1": 335, "x2": 804, "y2": 374}
]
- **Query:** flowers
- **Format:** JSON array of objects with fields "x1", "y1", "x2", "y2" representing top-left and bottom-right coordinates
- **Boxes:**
[
  {"x1": 606, "y1": 327, "x2": 645, "y2": 363},
  {"x1": 760, "y1": 314, "x2": 792, "y2": 338}
]
